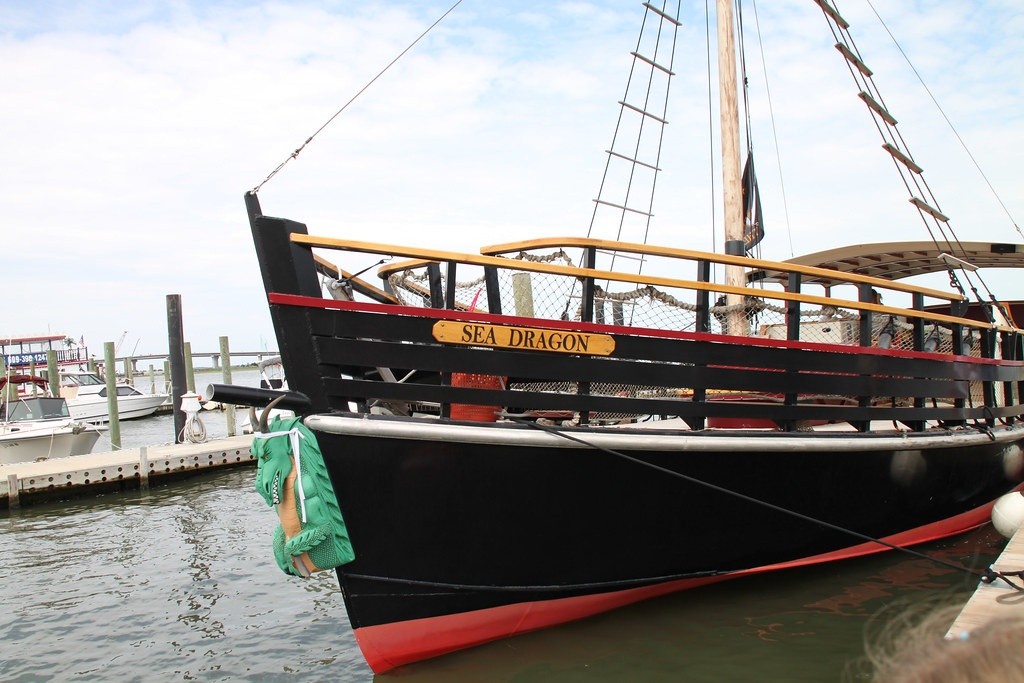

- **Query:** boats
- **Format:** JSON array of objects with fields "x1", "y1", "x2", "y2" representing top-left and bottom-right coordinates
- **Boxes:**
[
  {"x1": 0, "y1": 375, "x2": 110, "y2": 463},
  {"x1": 58, "y1": 371, "x2": 169, "y2": 423}
]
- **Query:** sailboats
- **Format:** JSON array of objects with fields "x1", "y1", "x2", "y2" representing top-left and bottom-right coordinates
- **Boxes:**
[{"x1": 205, "y1": 1, "x2": 1024, "y2": 678}]
